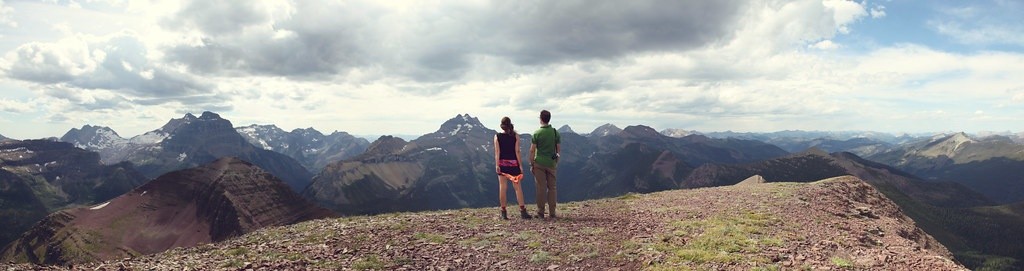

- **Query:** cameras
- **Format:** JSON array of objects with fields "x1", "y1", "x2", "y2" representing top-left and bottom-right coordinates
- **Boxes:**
[{"x1": 552, "y1": 153, "x2": 558, "y2": 160}]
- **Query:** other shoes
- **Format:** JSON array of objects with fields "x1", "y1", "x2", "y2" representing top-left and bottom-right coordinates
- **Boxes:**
[
  {"x1": 549, "y1": 214, "x2": 556, "y2": 218},
  {"x1": 534, "y1": 215, "x2": 544, "y2": 219}
]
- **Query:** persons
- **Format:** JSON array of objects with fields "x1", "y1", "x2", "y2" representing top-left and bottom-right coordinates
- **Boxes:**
[
  {"x1": 530, "y1": 110, "x2": 561, "y2": 218},
  {"x1": 493, "y1": 116, "x2": 531, "y2": 218}
]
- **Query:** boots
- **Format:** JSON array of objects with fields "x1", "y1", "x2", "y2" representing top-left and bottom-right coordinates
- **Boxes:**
[
  {"x1": 500, "y1": 210, "x2": 507, "y2": 219},
  {"x1": 519, "y1": 208, "x2": 532, "y2": 219}
]
